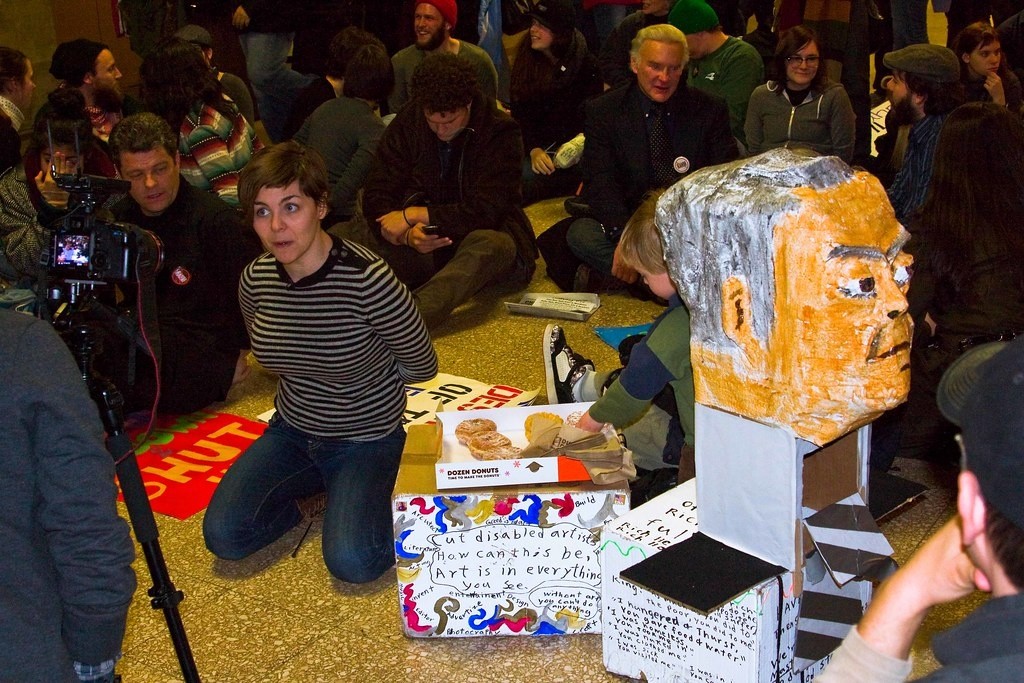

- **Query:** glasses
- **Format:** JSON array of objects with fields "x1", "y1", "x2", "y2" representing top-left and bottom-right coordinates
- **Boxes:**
[{"x1": 787, "y1": 56, "x2": 821, "y2": 66}]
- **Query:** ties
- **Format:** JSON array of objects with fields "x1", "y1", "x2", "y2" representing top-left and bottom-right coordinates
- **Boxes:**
[{"x1": 649, "y1": 102, "x2": 678, "y2": 186}]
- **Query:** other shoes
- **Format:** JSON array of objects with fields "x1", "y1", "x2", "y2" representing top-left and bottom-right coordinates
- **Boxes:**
[{"x1": 574, "y1": 262, "x2": 634, "y2": 296}]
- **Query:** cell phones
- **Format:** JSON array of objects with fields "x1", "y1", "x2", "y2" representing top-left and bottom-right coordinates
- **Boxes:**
[{"x1": 423, "y1": 225, "x2": 447, "y2": 239}]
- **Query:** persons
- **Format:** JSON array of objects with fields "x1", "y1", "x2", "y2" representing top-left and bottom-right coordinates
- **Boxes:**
[
  {"x1": 0, "y1": 309, "x2": 138, "y2": 683},
  {"x1": 203, "y1": 142, "x2": 439, "y2": 585},
  {"x1": 0, "y1": 1, "x2": 1022, "y2": 506},
  {"x1": 811, "y1": 338, "x2": 1023, "y2": 683}
]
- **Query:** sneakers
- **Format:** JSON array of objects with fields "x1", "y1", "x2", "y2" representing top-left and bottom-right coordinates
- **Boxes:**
[{"x1": 543, "y1": 324, "x2": 595, "y2": 404}]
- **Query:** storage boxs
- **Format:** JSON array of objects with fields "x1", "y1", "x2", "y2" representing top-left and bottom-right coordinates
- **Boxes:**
[{"x1": 390, "y1": 401, "x2": 897, "y2": 683}]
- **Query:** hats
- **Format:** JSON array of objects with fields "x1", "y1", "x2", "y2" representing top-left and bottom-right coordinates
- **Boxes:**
[
  {"x1": 531, "y1": 0, "x2": 576, "y2": 35},
  {"x1": 883, "y1": 43, "x2": 961, "y2": 84},
  {"x1": 50, "y1": 37, "x2": 109, "y2": 86},
  {"x1": 176, "y1": 25, "x2": 212, "y2": 48},
  {"x1": 667, "y1": 0, "x2": 719, "y2": 35},
  {"x1": 937, "y1": 335, "x2": 1024, "y2": 531},
  {"x1": 414, "y1": 0, "x2": 457, "y2": 31}
]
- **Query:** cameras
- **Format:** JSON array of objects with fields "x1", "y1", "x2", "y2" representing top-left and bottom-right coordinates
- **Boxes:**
[{"x1": 43, "y1": 217, "x2": 164, "y2": 285}]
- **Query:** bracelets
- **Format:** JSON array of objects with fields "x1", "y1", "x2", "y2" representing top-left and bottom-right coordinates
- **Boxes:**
[
  {"x1": 407, "y1": 229, "x2": 410, "y2": 247},
  {"x1": 402, "y1": 208, "x2": 413, "y2": 229}
]
